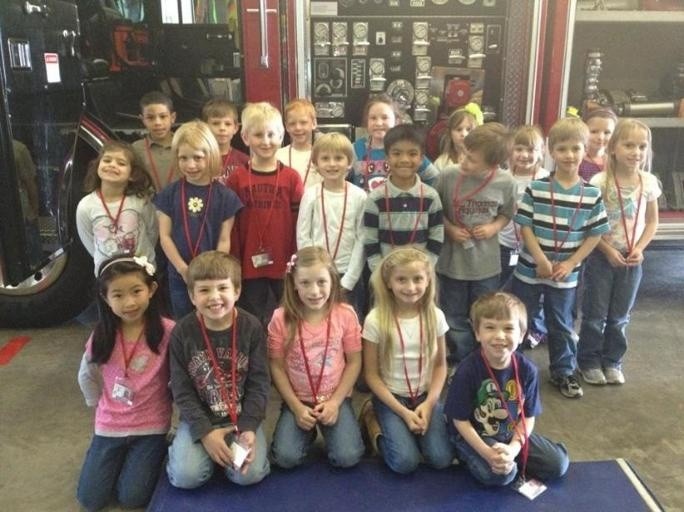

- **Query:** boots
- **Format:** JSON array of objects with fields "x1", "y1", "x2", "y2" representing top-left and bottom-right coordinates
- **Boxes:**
[{"x1": 358, "y1": 398, "x2": 384, "y2": 456}]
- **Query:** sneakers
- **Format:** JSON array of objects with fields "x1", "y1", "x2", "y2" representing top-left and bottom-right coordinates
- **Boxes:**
[
  {"x1": 550, "y1": 375, "x2": 584, "y2": 398},
  {"x1": 578, "y1": 366, "x2": 624, "y2": 385},
  {"x1": 526, "y1": 329, "x2": 544, "y2": 349}
]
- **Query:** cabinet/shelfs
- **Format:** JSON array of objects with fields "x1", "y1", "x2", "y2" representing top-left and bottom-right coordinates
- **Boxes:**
[{"x1": 551, "y1": 0, "x2": 684, "y2": 244}]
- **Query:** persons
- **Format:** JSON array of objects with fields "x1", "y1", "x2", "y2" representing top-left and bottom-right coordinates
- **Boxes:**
[
  {"x1": 76, "y1": 92, "x2": 662, "y2": 401},
  {"x1": 262, "y1": 242, "x2": 369, "y2": 472},
  {"x1": 354, "y1": 245, "x2": 456, "y2": 476},
  {"x1": 442, "y1": 291, "x2": 572, "y2": 491},
  {"x1": 163, "y1": 246, "x2": 273, "y2": 490},
  {"x1": 11, "y1": 138, "x2": 43, "y2": 269},
  {"x1": 73, "y1": 255, "x2": 176, "y2": 512}
]
